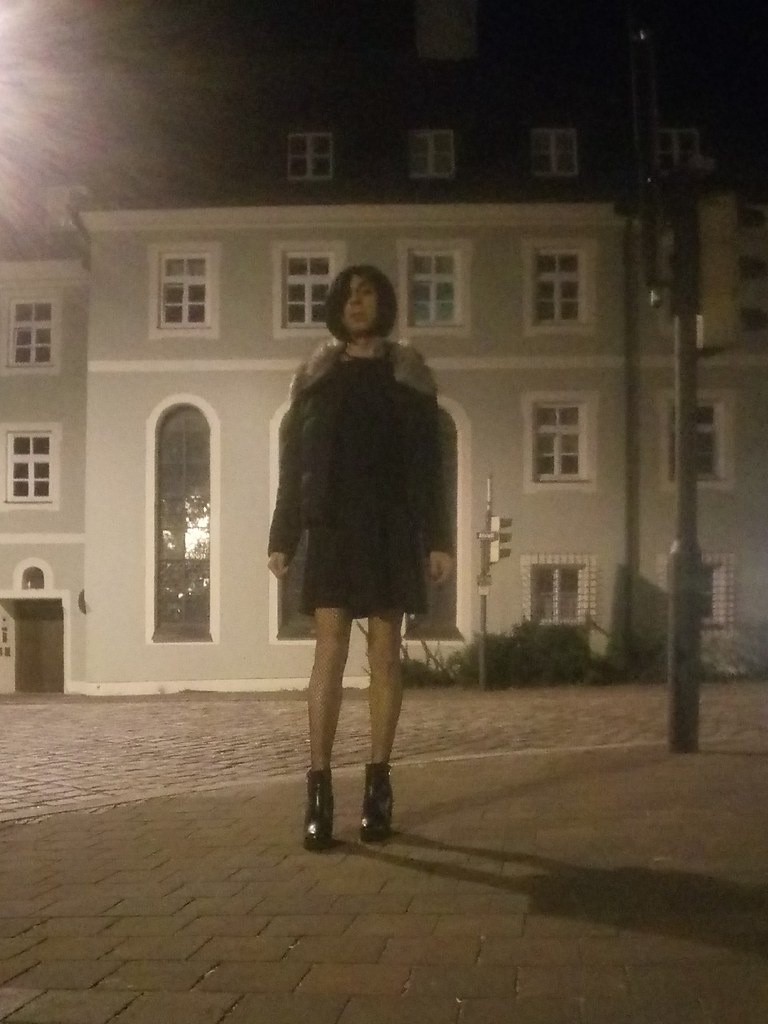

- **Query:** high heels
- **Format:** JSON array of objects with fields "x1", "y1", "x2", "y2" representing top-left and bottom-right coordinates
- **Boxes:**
[
  {"x1": 302, "y1": 768, "x2": 334, "y2": 849},
  {"x1": 359, "y1": 762, "x2": 394, "y2": 841}
]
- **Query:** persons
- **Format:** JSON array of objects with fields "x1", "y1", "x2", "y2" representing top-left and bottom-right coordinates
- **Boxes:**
[{"x1": 269, "y1": 266, "x2": 457, "y2": 852}]
